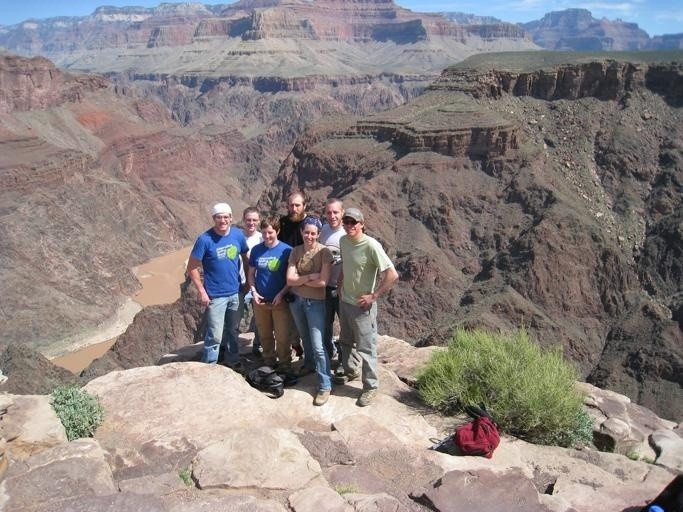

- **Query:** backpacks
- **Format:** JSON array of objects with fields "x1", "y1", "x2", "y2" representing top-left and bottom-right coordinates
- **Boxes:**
[
  {"x1": 451, "y1": 407, "x2": 500, "y2": 459},
  {"x1": 243, "y1": 367, "x2": 297, "y2": 398}
]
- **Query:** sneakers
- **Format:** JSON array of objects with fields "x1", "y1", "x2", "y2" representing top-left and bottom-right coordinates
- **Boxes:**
[
  {"x1": 312, "y1": 388, "x2": 332, "y2": 406},
  {"x1": 228, "y1": 361, "x2": 245, "y2": 371},
  {"x1": 295, "y1": 367, "x2": 312, "y2": 377},
  {"x1": 333, "y1": 371, "x2": 359, "y2": 385},
  {"x1": 357, "y1": 388, "x2": 377, "y2": 407},
  {"x1": 334, "y1": 362, "x2": 344, "y2": 376},
  {"x1": 289, "y1": 343, "x2": 302, "y2": 361},
  {"x1": 252, "y1": 344, "x2": 261, "y2": 357}
]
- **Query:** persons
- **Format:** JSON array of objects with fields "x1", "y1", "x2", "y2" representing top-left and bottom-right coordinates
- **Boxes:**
[
  {"x1": 278, "y1": 191, "x2": 309, "y2": 357},
  {"x1": 187, "y1": 203, "x2": 249, "y2": 368},
  {"x1": 237, "y1": 207, "x2": 265, "y2": 356},
  {"x1": 319, "y1": 198, "x2": 347, "y2": 374},
  {"x1": 247, "y1": 217, "x2": 297, "y2": 386},
  {"x1": 333, "y1": 208, "x2": 399, "y2": 407},
  {"x1": 285, "y1": 214, "x2": 333, "y2": 406}
]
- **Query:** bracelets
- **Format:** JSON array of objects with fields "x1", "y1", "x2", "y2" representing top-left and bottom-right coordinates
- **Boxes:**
[{"x1": 250, "y1": 290, "x2": 255, "y2": 296}]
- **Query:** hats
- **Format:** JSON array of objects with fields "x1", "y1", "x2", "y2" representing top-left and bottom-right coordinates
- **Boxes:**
[
  {"x1": 340, "y1": 208, "x2": 365, "y2": 223},
  {"x1": 211, "y1": 203, "x2": 232, "y2": 217},
  {"x1": 303, "y1": 215, "x2": 322, "y2": 229}
]
